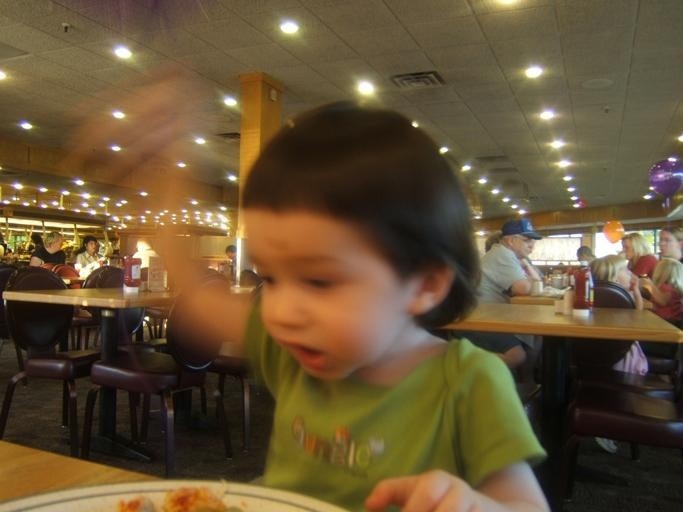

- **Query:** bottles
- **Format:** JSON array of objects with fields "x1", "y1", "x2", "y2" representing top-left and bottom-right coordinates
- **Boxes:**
[
  {"x1": 572, "y1": 268, "x2": 589, "y2": 319},
  {"x1": 562, "y1": 288, "x2": 573, "y2": 314},
  {"x1": 119, "y1": 257, "x2": 142, "y2": 297},
  {"x1": 578, "y1": 260, "x2": 594, "y2": 315}
]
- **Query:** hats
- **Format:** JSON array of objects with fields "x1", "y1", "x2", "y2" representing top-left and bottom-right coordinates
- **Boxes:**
[{"x1": 501, "y1": 218, "x2": 541, "y2": 239}]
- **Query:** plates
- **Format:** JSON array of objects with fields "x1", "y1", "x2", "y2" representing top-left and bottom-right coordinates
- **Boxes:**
[{"x1": 0, "y1": 479, "x2": 349, "y2": 512}]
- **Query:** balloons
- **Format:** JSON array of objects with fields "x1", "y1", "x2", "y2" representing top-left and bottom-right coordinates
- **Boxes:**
[{"x1": 649, "y1": 158, "x2": 682, "y2": 198}]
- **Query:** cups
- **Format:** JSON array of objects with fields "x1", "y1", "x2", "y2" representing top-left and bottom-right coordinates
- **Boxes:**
[
  {"x1": 530, "y1": 269, "x2": 575, "y2": 298},
  {"x1": 553, "y1": 300, "x2": 562, "y2": 313}
]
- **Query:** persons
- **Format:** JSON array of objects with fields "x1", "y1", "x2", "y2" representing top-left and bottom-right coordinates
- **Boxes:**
[
  {"x1": 457, "y1": 218, "x2": 682, "y2": 387},
  {"x1": 29, "y1": 233, "x2": 236, "y2": 351},
  {"x1": 75, "y1": 94, "x2": 552, "y2": 510}
]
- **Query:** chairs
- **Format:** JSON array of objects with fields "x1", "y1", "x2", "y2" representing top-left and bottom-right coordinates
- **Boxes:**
[
  {"x1": 440, "y1": 274, "x2": 682, "y2": 511},
  {"x1": 0, "y1": 256, "x2": 268, "y2": 468}
]
